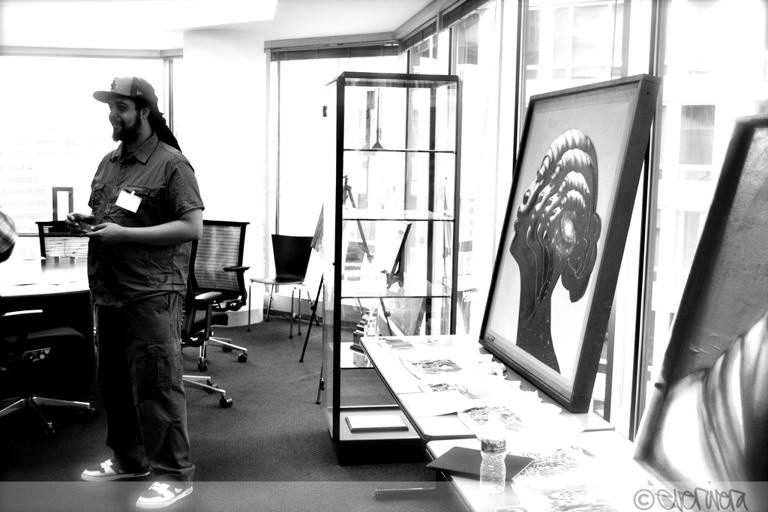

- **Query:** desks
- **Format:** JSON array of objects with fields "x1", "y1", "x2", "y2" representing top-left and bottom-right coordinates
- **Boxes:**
[
  {"x1": 0, "y1": 233, "x2": 101, "y2": 418},
  {"x1": 356, "y1": 328, "x2": 686, "y2": 512}
]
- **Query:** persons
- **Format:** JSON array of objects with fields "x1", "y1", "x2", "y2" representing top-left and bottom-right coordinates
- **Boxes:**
[{"x1": 64, "y1": 75, "x2": 204, "y2": 509}]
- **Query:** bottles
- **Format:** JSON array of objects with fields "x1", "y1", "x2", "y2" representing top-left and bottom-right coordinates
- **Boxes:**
[{"x1": 478, "y1": 412, "x2": 507, "y2": 494}]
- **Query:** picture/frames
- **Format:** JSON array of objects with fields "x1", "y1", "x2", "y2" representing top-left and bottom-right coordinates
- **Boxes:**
[
  {"x1": 631, "y1": 111, "x2": 768, "y2": 512},
  {"x1": 477, "y1": 72, "x2": 660, "y2": 416}
]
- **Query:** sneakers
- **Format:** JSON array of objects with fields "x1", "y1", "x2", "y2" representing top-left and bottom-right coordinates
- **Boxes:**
[
  {"x1": 136, "y1": 481, "x2": 193, "y2": 509},
  {"x1": 81, "y1": 456, "x2": 151, "y2": 482}
]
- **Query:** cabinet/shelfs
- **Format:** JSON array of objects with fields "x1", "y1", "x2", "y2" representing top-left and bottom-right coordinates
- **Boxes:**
[{"x1": 319, "y1": 68, "x2": 469, "y2": 465}]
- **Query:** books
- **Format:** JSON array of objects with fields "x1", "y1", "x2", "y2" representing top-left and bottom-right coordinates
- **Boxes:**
[{"x1": 427, "y1": 446, "x2": 535, "y2": 482}]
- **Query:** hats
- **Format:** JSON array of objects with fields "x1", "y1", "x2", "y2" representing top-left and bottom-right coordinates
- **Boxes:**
[{"x1": 93, "y1": 76, "x2": 158, "y2": 106}]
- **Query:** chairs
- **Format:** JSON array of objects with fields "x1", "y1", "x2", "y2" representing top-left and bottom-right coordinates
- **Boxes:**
[
  {"x1": 179, "y1": 220, "x2": 251, "y2": 371},
  {"x1": 247, "y1": 234, "x2": 318, "y2": 341}
]
